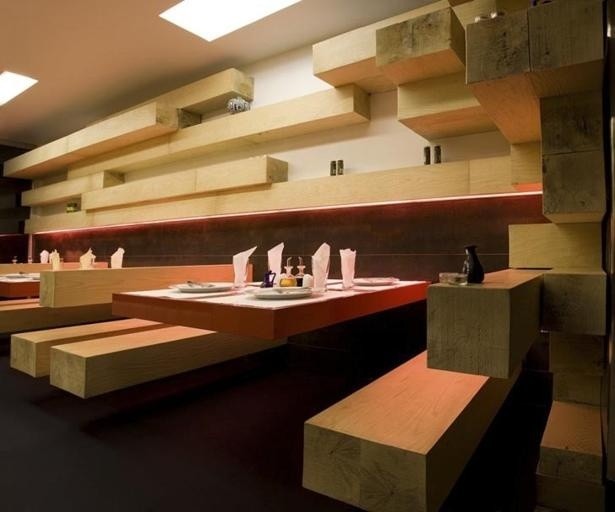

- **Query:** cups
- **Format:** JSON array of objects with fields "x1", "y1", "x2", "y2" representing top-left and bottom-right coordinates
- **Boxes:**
[
  {"x1": 340, "y1": 256, "x2": 356, "y2": 290},
  {"x1": 267, "y1": 255, "x2": 282, "y2": 286},
  {"x1": 310, "y1": 255, "x2": 330, "y2": 293},
  {"x1": 231, "y1": 257, "x2": 249, "y2": 289}
]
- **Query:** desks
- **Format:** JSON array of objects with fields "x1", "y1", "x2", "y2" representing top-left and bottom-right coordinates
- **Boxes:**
[{"x1": 112, "y1": 268, "x2": 430, "y2": 342}]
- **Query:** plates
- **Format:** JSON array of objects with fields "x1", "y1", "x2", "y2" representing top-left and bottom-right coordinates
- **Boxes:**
[
  {"x1": 172, "y1": 282, "x2": 231, "y2": 293},
  {"x1": 355, "y1": 278, "x2": 398, "y2": 286},
  {"x1": 254, "y1": 286, "x2": 312, "y2": 299}
]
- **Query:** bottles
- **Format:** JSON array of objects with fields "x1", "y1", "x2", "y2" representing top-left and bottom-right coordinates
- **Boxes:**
[
  {"x1": 281, "y1": 265, "x2": 307, "y2": 287},
  {"x1": 330, "y1": 160, "x2": 343, "y2": 176},
  {"x1": 463, "y1": 245, "x2": 485, "y2": 284},
  {"x1": 423, "y1": 144, "x2": 441, "y2": 164}
]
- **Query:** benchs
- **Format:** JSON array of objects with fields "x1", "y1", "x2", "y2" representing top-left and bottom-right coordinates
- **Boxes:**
[
  {"x1": 10, "y1": 313, "x2": 294, "y2": 398},
  {"x1": 301, "y1": 353, "x2": 544, "y2": 511}
]
